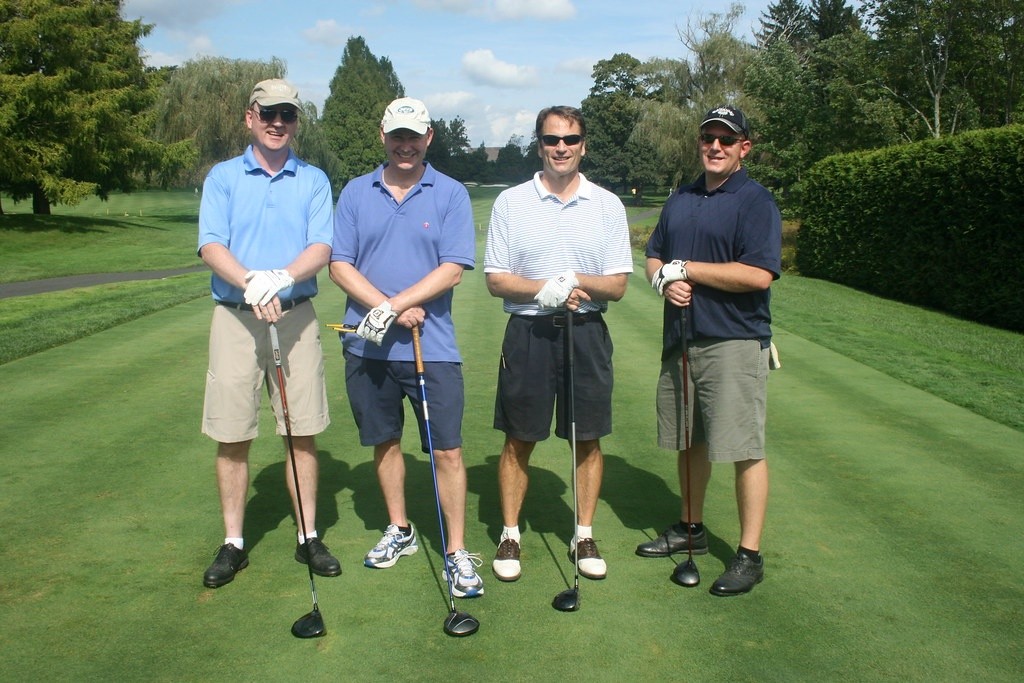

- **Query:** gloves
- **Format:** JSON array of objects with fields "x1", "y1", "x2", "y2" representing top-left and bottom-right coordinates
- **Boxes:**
[
  {"x1": 534, "y1": 270, "x2": 579, "y2": 310},
  {"x1": 652, "y1": 259, "x2": 692, "y2": 296},
  {"x1": 243, "y1": 268, "x2": 295, "y2": 306},
  {"x1": 356, "y1": 300, "x2": 396, "y2": 347}
]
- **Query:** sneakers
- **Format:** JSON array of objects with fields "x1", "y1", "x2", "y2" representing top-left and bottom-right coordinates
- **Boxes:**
[
  {"x1": 442, "y1": 549, "x2": 485, "y2": 597},
  {"x1": 363, "y1": 523, "x2": 419, "y2": 568}
]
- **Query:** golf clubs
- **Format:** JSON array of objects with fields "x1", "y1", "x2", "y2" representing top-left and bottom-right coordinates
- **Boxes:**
[
  {"x1": 266, "y1": 321, "x2": 326, "y2": 640},
  {"x1": 673, "y1": 305, "x2": 701, "y2": 588},
  {"x1": 551, "y1": 308, "x2": 580, "y2": 612},
  {"x1": 410, "y1": 322, "x2": 482, "y2": 637}
]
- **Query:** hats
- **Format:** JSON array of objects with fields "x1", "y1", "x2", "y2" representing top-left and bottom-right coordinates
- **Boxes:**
[
  {"x1": 382, "y1": 97, "x2": 431, "y2": 135},
  {"x1": 250, "y1": 78, "x2": 303, "y2": 113},
  {"x1": 698, "y1": 104, "x2": 749, "y2": 139}
]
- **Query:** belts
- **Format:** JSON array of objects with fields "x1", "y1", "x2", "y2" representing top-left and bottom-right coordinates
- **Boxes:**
[
  {"x1": 533, "y1": 311, "x2": 601, "y2": 327},
  {"x1": 219, "y1": 296, "x2": 311, "y2": 313}
]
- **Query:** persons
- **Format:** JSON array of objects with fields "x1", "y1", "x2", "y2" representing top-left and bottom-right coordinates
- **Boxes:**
[
  {"x1": 197, "y1": 78, "x2": 341, "y2": 589},
  {"x1": 635, "y1": 104, "x2": 782, "y2": 596},
  {"x1": 330, "y1": 95, "x2": 484, "y2": 599},
  {"x1": 484, "y1": 107, "x2": 633, "y2": 584}
]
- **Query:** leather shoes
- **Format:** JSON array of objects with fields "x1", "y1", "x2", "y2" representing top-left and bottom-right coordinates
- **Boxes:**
[
  {"x1": 569, "y1": 538, "x2": 607, "y2": 579},
  {"x1": 636, "y1": 527, "x2": 708, "y2": 557},
  {"x1": 709, "y1": 553, "x2": 766, "y2": 597},
  {"x1": 203, "y1": 542, "x2": 247, "y2": 588},
  {"x1": 492, "y1": 530, "x2": 522, "y2": 581},
  {"x1": 295, "y1": 537, "x2": 342, "y2": 577}
]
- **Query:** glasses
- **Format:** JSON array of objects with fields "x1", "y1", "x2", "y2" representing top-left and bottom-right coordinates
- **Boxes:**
[
  {"x1": 251, "y1": 107, "x2": 298, "y2": 123},
  {"x1": 325, "y1": 322, "x2": 360, "y2": 334},
  {"x1": 699, "y1": 133, "x2": 745, "y2": 146},
  {"x1": 541, "y1": 134, "x2": 586, "y2": 147}
]
